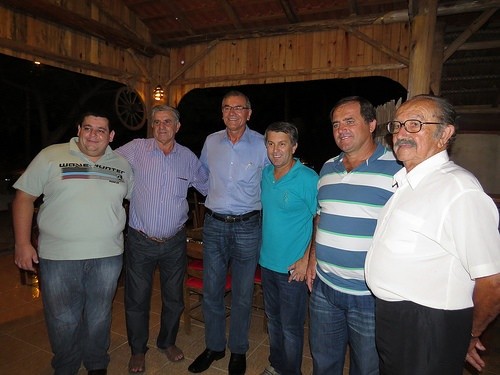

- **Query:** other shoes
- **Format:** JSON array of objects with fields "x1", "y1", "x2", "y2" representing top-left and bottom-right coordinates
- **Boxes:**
[
  {"x1": 88, "y1": 369, "x2": 107, "y2": 375},
  {"x1": 263, "y1": 365, "x2": 279, "y2": 375}
]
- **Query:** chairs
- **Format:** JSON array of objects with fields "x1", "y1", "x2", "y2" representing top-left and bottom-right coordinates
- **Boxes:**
[{"x1": 183, "y1": 227, "x2": 268, "y2": 334}]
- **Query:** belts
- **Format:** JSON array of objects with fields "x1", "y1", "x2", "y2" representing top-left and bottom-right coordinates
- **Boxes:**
[
  {"x1": 205, "y1": 208, "x2": 260, "y2": 225},
  {"x1": 137, "y1": 230, "x2": 175, "y2": 243}
]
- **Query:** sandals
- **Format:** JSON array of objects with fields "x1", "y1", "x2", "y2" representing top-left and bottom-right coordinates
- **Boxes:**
[
  {"x1": 129, "y1": 352, "x2": 145, "y2": 375},
  {"x1": 158, "y1": 345, "x2": 184, "y2": 362}
]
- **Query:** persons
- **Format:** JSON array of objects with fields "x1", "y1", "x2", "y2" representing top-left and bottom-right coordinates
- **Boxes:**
[
  {"x1": 12, "y1": 111, "x2": 134, "y2": 375},
  {"x1": 364, "y1": 93, "x2": 500, "y2": 375},
  {"x1": 306, "y1": 95, "x2": 404, "y2": 375},
  {"x1": 184, "y1": 90, "x2": 271, "y2": 375},
  {"x1": 113, "y1": 105, "x2": 210, "y2": 375},
  {"x1": 259, "y1": 121, "x2": 321, "y2": 375}
]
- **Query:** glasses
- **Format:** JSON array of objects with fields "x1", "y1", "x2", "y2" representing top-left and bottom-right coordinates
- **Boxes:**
[
  {"x1": 222, "y1": 105, "x2": 250, "y2": 113},
  {"x1": 386, "y1": 120, "x2": 443, "y2": 134}
]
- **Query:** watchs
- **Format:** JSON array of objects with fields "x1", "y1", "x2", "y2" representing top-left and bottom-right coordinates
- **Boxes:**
[{"x1": 470, "y1": 331, "x2": 481, "y2": 340}]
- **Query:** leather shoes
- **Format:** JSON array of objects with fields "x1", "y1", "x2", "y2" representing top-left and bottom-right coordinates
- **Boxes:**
[
  {"x1": 229, "y1": 353, "x2": 246, "y2": 375},
  {"x1": 188, "y1": 348, "x2": 225, "y2": 373}
]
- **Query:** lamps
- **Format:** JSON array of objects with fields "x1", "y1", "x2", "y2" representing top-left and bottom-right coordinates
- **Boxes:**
[{"x1": 153, "y1": 56, "x2": 164, "y2": 101}]
